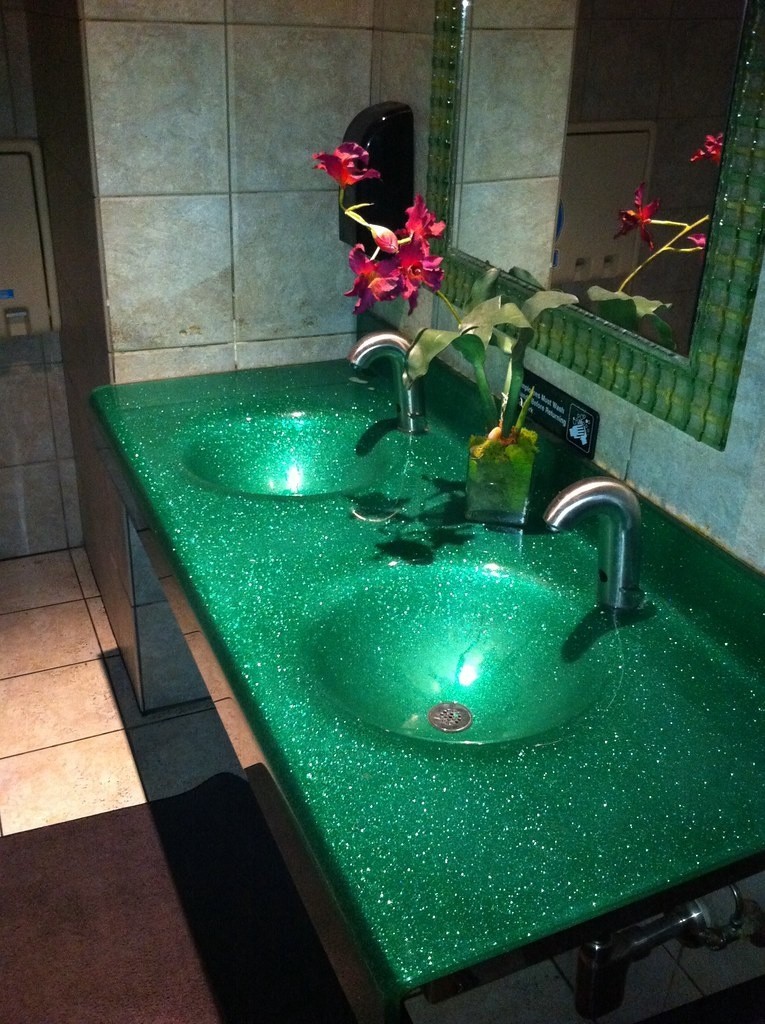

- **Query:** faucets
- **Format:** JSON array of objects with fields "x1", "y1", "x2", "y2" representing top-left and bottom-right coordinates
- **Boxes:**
[
  {"x1": 539, "y1": 472, "x2": 646, "y2": 614},
  {"x1": 343, "y1": 326, "x2": 429, "y2": 438},
  {"x1": 294, "y1": 560, "x2": 627, "y2": 759}
]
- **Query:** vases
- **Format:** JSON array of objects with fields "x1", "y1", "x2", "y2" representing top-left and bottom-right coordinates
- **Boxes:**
[{"x1": 465, "y1": 432, "x2": 541, "y2": 528}]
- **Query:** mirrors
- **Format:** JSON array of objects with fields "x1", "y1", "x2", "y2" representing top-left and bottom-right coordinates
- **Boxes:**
[{"x1": 420, "y1": 0, "x2": 765, "y2": 452}]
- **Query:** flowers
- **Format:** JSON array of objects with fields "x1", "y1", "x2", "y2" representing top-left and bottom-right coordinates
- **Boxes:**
[
  {"x1": 580, "y1": 134, "x2": 724, "y2": 350},
  {"x1": 311, "y1": 143, "x2": 580, "y2": 443}
]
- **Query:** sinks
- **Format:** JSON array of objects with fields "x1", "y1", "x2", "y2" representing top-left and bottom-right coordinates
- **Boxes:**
[{"x1": 175, "y1": 412, "x2": 397, "y2": 502}]
